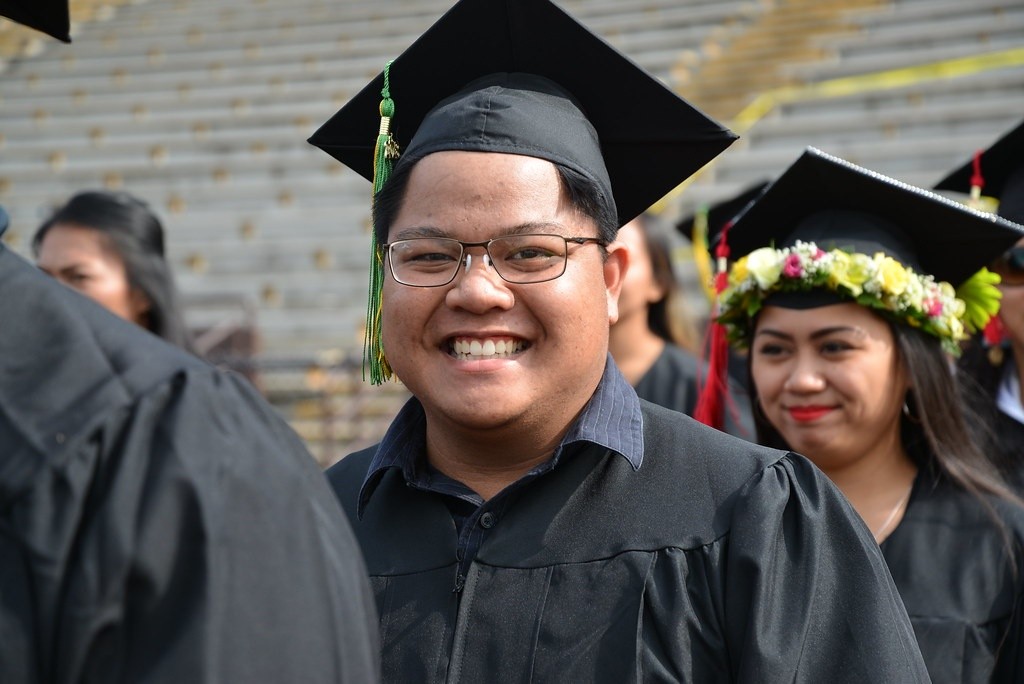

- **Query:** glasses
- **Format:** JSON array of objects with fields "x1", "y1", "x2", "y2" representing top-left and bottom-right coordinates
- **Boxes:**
[{"x1": 382, "y1": 234, "x2": 609, "y2": 288}]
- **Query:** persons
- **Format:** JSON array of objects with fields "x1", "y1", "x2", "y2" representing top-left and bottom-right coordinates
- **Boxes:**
[
  {"x1": 603, "y1": 213, "x2": 758, "y2": 446},
  {"x1": 30, "y1": 189, "x2": 201, "y2": 358},
  {"x1": 0, "y1": 0, "x2": 379, "y2": 683},
  {"x1": 307, "y1": 1, "x2": 935, "y2": 684},
  {"x1": 709, "y1": 148, "x2": 1024, "y2": 684},
  {"x1": 673, "y1": 118, "x2": 1024, "y2": 495}
]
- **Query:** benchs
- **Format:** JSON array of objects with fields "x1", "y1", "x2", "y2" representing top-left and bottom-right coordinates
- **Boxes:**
[
  {"x1": 661, "y1": 0, "x2": 1023, "y2": 332},
  {"x1": 0, "y1": 0, "x2": 777, "y2": 401}
]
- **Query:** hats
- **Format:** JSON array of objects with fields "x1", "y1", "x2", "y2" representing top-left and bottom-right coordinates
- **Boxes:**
[
  {"x1": 0, "y1": 0, "x2": 72, "y2": 43},
  {"x1": 307, "y1": 0, "x2": 740, "y2": 386},
  {"x1": 674, "y1": 121, "x2": 1024, "y2": 439}
]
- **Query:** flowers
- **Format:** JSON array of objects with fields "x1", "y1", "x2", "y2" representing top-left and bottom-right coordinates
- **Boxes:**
[{"x1": 718, "y1": 238, "x2": 1005, "y2": 358}]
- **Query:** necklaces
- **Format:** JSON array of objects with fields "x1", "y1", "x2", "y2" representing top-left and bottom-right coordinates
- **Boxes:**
[{"x1": 873, "y1": 486, "x2": 912, "y2": 540}]
